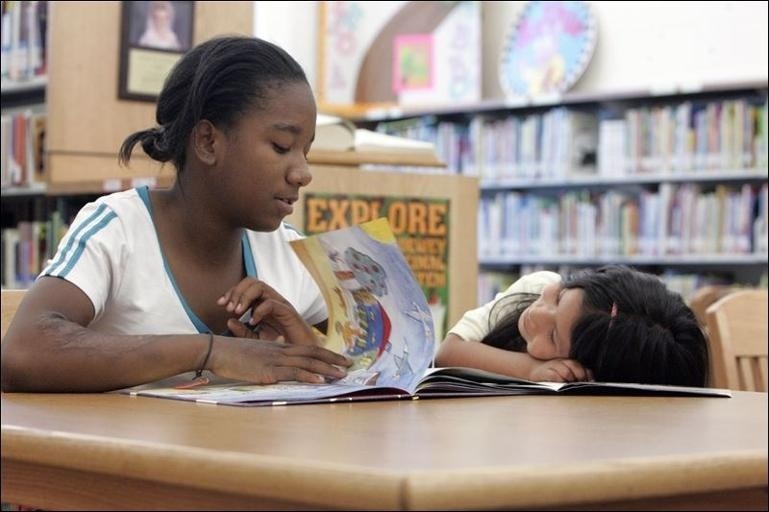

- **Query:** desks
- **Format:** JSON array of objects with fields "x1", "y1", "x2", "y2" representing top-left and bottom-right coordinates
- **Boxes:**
[{"x1": 1, "y1": 378, "x2": 768, "y2": 509}]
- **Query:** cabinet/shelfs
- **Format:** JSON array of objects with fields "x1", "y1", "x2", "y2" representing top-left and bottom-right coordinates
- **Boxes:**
[
  {"x1": 362, "y1": 79, "x2": 767, "y2": 313},
  {"x1": 1, "y1": 1, "x2": 257, "y2": 288}
]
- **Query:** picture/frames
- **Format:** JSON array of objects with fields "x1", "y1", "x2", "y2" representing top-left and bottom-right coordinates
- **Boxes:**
[{"x1": 118, "y1": 0, "x2": 197, "y2": 103}]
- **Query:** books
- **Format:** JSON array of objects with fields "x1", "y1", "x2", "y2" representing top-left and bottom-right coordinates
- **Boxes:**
[
  {"x1": 125, "y1": 217, "x2": 546, "y2": 407},
  {"x1": 1, "y1": 0, "x2": 73, "y2": 290},
  {"x1": 473, "y1": 180, "x2": 769, "y2": 256},
  {"x1": 486, "y1": 360, "x2": 732, "y2": 398},
  {"x1": 658, "y1": 272, "x2": 734, "y2": 310},
  {"x1": 478, "y1": 266, "x2": 573, "y2": 307},
  {"x1": 364, "y1": 94, "x2": 768, "y2": 178}
]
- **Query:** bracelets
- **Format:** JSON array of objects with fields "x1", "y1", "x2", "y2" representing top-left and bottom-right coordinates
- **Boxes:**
[{"x1": 190, "y1": 328, "x2": 219, "y2": 380}]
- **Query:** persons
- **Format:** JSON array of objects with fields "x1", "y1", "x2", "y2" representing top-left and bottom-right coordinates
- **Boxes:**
[
  {"x1": 428, "y1": 257, "x2": 717, "y2": 390},
  {"x1": 2, "y1": 34, "x2": 354, "y2": 388},
  {"x1": 134, "y1": 2, "x2": 182, "y2": 52}
]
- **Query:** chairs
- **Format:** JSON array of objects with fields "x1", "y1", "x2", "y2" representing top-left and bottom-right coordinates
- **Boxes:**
[{"x1": 705, "y1": 290, "x2": 769, "y2": 393}]
